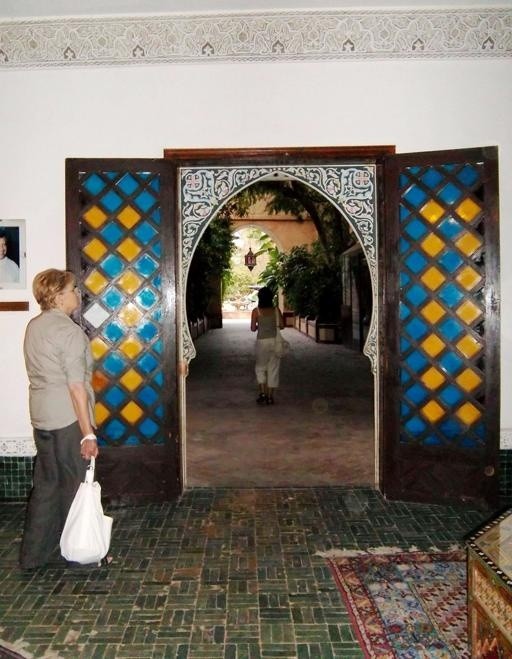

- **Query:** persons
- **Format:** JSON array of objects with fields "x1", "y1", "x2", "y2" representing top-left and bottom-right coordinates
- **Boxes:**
[
  {"x1": 251, "y1": 286, "x2": 286, "y2": 404},
  {"x1": 0, "y1": 230, "x2": 20, "y2": 285},
  {"x1": 18, "y1": 268, "x2": 114, "y2": 571}
]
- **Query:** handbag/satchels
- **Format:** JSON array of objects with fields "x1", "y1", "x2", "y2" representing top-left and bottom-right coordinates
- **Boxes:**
[
  {"x1": 59, "y1": 434, "x2": 114, "y2": 564},
  {"x1": 274, "y1": 307, "x2": 291, "y2": 359}
]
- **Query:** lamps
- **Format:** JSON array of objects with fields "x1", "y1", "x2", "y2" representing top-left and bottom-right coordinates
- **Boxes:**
[{"x1": 245, "y1": 225, "x2": 256, "y2": 271}]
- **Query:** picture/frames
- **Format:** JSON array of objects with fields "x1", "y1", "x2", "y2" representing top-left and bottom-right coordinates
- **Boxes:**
[{"x1": 0, "y1": 219, "x2": 28, "y2": 291}]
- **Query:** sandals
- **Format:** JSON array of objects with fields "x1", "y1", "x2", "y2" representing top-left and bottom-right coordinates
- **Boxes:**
[{"x1": 69, "y1": 556, "x2": 114, "y2": 575}]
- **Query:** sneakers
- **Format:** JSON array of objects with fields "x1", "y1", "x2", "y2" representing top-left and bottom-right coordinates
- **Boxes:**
[
  {"x1": 266, "y1": 398, "x2": 274, "y2": 405},
  {"x1": 256, "y1": 393, "x2": 266, "y2": 402}
]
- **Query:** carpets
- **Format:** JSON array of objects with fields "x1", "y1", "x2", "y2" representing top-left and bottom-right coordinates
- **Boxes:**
[{"x1": 312, "y1": 541, "x2": 477, "y2": 659}]
